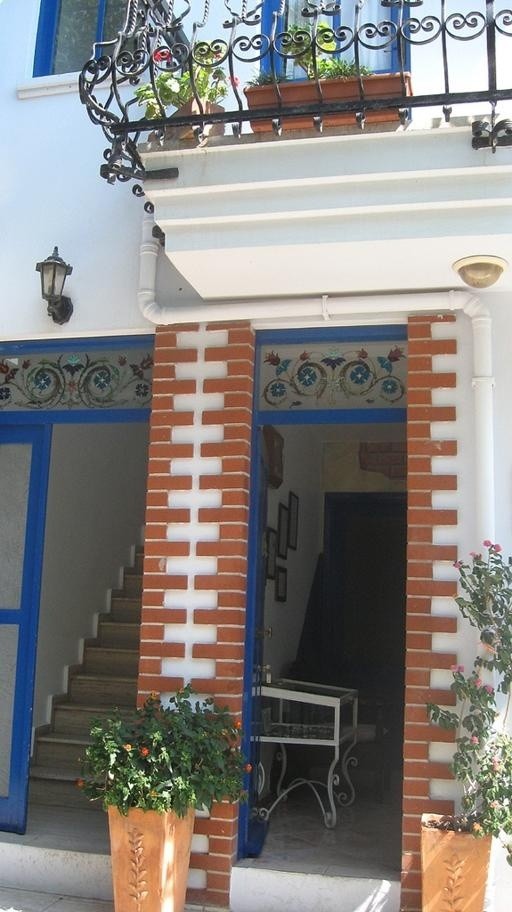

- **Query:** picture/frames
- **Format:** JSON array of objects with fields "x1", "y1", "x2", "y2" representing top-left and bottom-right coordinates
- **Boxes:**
[{"x1": 266, "y1": 490, "x2": 300, "y2": 604}]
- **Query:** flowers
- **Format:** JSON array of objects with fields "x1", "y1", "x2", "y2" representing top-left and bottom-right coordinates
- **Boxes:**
[
  {"x1": 134, "y1": 39, "x2": 239, "y2": 121},
  {"x1": 74, "y1": 683, "x2": 252, "y2": 818},
  {"x1": 425, "y1": 538, "x2": 511, "y2": 868}
]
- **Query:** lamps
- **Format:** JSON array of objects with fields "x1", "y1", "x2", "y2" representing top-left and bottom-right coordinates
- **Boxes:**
[{"x1": 35, "y1": 245, "x2": 74, "y2": 325}]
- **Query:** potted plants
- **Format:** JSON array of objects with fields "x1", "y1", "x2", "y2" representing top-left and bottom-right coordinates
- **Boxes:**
[{"x1": 244, "y1": 19, "x2": 412, "y2": 132}]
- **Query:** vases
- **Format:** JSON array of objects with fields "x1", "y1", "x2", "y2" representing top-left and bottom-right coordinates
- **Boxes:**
[
  {"x1": 419, "y1": 813, "x2": 492, "y2": 912},
  {"x1": 147, "y1": 98, "x2": 225, "y2": 141},
  {"x1": 104, "y1": 804, "x2": 196, "y2": 912}
]
